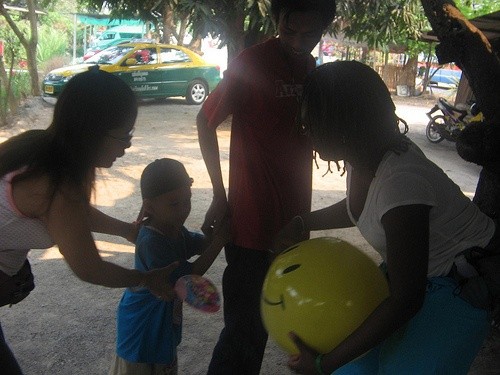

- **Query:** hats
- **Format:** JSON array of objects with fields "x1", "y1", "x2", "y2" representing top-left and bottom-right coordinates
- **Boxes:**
[{"x1": 137, "y1": 158, "x2": 189, "y2": 221}]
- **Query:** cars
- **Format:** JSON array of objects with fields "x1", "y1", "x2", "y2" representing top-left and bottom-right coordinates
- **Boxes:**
[{"x1": 41, "y1": 42, "x2": 221, "y2": 105}]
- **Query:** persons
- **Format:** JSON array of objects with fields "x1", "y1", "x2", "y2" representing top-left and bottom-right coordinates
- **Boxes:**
[
  {"x1": 118, "y1": 158, "x2": 233, "y2": 373},
  {"x1": 0, "y1": 66, "x2": 174, "y2": 374},
  {"x1": 195, "y1": 0, "x2": 335, "y2": 374},
  {"x1": 285, "y1": 60, "x2": 500, "y2": 374}
]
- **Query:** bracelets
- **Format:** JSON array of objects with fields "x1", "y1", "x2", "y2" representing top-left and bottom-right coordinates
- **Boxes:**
[
  {"x1": 315, "y1": 353, "x2": 324, "y2": 374},
  {"x1": 293, "y1": 215, "x2": 305, "y2": 235}
]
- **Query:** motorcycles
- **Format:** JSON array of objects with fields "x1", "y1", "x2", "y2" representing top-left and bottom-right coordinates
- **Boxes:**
[{"x1": 425, "y1": 97, "x2": 484, "y2": 143}]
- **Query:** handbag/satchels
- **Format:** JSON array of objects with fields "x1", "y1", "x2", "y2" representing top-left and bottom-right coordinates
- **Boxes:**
[
  {"x1": 0, "y1": 258, "x2": 35, "y2": 308},
  {"x1": 451, "y1": 247, "x2": 500, "y2": 310}
]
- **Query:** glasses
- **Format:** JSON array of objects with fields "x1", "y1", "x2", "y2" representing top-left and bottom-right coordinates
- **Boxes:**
[{"x1": 107, "y1": 128, "x2": 137, "y2": 143}]
